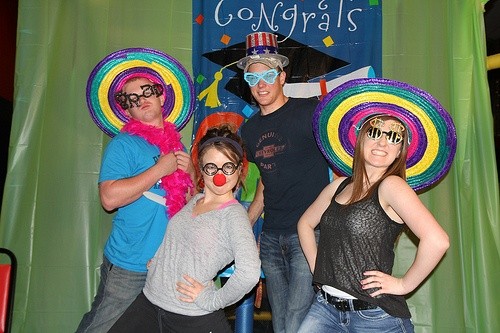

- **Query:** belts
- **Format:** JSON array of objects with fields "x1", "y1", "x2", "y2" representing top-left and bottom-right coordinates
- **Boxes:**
[{"x1": 320, "y1": 288, "x2": 376, "y2": 311}]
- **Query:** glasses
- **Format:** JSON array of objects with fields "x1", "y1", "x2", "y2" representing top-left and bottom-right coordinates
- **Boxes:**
[
  {"x1": 243, "y1": 69, "x2": 283, "y2": 86},
  {"x1": 367, "y1": 128, "x2": 404, "y2": 144},
  {"x1": 202, "y1": 160, "x2": 240, "y2": 176}
]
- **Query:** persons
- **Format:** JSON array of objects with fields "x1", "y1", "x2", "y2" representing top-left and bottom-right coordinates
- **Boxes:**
[
  {"x1": 76, "y1": 48, "x2": 196, "y2": 333},
  {"x1": 297, "y1": 78, "x2": 457, "y2": 333},
  {"x1": 107, "y1": 124, "x2": 262, "y2": 333},
  {"x1": 238, "y1": 32, "x2": 330, "y2": 333}
]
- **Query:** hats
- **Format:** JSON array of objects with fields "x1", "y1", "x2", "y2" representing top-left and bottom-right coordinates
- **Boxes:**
[
  {"x1": 237, "y1": 32, "x2": 290, "y2": 73},
  {"x1": 190, "y1": 112, "x2": 250, "y2": 188},
  {"x1": 85, "y1": 48, "x2": 195, "y2": 138},
  {"x1": 313, "y1": 78, "x2": 457, "y2": 190}
]
